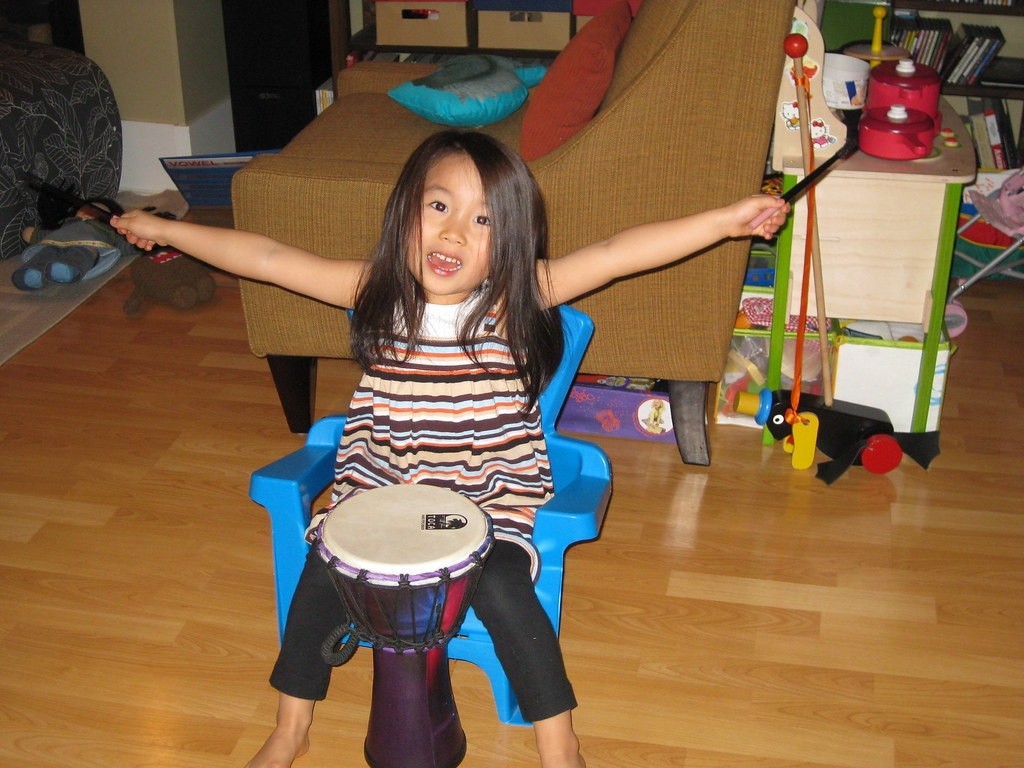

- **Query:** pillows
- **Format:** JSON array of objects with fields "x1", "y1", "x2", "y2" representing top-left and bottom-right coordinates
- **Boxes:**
[
  {"x1": 387, "y1": 56, "x2": 551, "y2": 129},
  {"x1": 519, "y1": 1, "x2": 633, "y2": 162}
]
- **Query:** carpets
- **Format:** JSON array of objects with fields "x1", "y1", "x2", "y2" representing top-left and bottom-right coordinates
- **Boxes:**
[{"x1": 0, "y1": 186, "x2": 190, "y2": 368}]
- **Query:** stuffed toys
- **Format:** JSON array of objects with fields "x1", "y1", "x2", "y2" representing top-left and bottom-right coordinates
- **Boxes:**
[
  {"x1": 14, "y1": 196, "x2": 143, "y2": 289},
  {"x1": 125, "y1": 209, "x2": 223, "y2": 310}
]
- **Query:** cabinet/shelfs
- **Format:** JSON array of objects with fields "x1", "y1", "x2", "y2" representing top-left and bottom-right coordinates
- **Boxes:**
[{"x1": 887, "y1": 0, "x2": 1024, "y2": 102}]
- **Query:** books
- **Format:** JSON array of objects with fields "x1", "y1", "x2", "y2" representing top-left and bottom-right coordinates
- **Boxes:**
[
  {"x1": 962, "y1": 98, "x2": 1019, "y2": 170},
  {"x1": 891, "y1": 17, "x2": 1024, "y2": 91},
  {"x1": 936, "y1": 0, "x2": 1013, "y2": 7}
]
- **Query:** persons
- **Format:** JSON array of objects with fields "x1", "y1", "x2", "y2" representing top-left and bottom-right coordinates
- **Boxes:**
[{"x1": 110, "y1": 128, "x2": 791, "y2": 768}]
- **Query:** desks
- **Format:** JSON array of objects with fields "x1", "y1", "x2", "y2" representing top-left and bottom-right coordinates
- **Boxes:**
[{"x1": 760, "y1": 0, "x2": 978, "y2": 450}]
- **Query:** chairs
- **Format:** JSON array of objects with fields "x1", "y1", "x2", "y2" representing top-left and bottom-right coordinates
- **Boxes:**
[
  {"x1": 249, "y1": 304, "x2": 614, "y2": 726},
  {"x1": 230, "y1": 0, "x2": 798, "y2": 466}
]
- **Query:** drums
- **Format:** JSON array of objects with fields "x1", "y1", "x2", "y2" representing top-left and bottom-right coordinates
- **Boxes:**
[{"x1": 304, "y1": 487, "x2": 494, "y2": 764}]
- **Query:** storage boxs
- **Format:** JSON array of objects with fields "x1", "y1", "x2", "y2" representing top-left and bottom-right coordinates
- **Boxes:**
[
  {"x1": 951, "y1": 167, "x2": 1024, "y2": 281},
  {"x1": 375, "y1": 1, "x2": 468, "y2": 48},
  {"x1": 475, "y1": 1, "x2": 643, "y2": 51},
  {"x1": 554, "y1": 372, "x2": 678, "y2": 445},
  {"x1": 706, "y1": 250, "x2": 952, "y2": 433}
]
